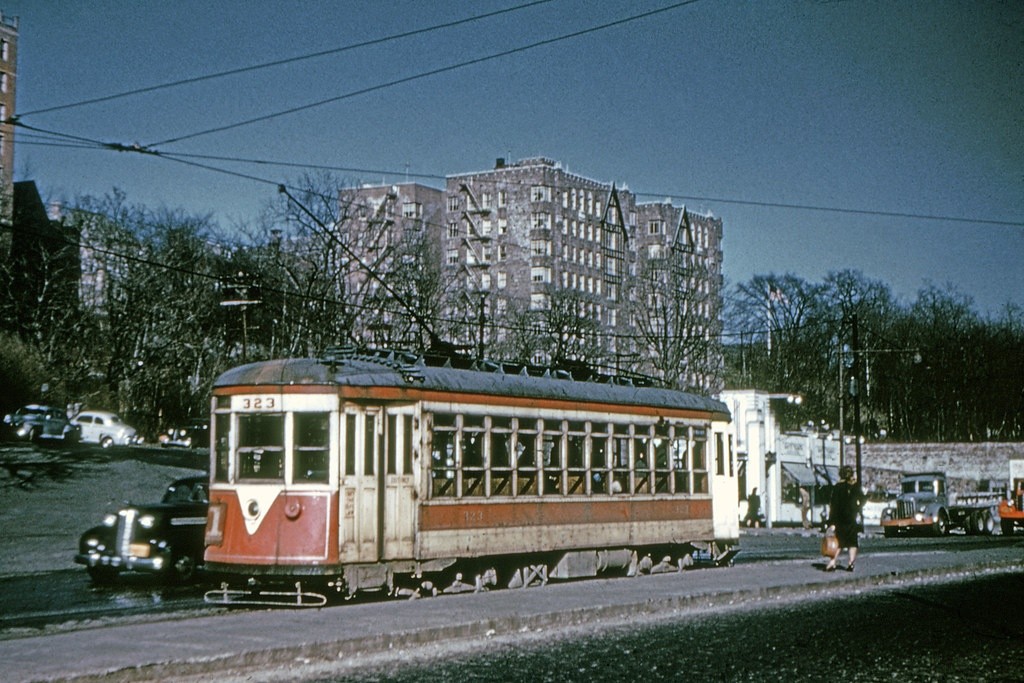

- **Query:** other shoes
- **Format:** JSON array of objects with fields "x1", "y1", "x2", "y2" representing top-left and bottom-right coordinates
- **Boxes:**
[
  {"x1": 822, "y1": 563, "x2": 838, "y2": 572},
  {"x1": 846, "y1": 564, "x2": 856, "y2": 573}
]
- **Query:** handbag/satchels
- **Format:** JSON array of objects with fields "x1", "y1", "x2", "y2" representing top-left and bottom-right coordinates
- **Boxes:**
[{"x1": 821, "y1": 528, "x2": 839, "y2": 558}]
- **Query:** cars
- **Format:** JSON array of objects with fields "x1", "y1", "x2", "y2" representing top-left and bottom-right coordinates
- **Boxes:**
[
  {"x1": 3, "y1": 404, "x2": 66, "y2": 441},
  {"x1": 61, "y1": 412, "x2": 146, "y2": 448},
  {"x1": 159, "y1": 416, "x2": 210, "y2": 449},
  {"x1": 73, "y1": 476, "x2": 208, "y2": 584}
]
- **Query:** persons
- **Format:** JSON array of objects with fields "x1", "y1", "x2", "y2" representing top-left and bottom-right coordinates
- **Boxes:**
[
  {"x1": 799, "y1": 486, "x2": 810, "y2": 530},
  {"x1": 823, "y1": 465, "x2": 867, "y2": 572},
  {"x1": 744, "y1": 488, "x2": 760, "y2": 529},
  {"x1": 603, "y1": 470, "x2": 622, "y2": 493}
]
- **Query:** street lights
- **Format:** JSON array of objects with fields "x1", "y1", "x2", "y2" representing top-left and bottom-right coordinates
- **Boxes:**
[{"x1": 834, "y1": 344, "x2": 928, "y2": 538}]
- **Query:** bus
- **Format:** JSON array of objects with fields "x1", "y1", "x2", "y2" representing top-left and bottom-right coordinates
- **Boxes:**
[{"x1": 205, "y1": 347, "x2": 739, "y2": 606}]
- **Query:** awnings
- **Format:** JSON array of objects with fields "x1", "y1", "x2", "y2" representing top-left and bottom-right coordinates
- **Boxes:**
[{"x1": 782, "y1": 463, "x2": 842, "y2": 489}]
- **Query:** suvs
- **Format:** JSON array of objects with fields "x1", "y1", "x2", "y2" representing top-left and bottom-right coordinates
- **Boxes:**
[{"x1": 999, "y1": 477, "x2": 1024, "y2": 537}]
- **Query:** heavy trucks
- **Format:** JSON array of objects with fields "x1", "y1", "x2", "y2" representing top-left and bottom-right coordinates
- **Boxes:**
[{"x1": 880, "y1": 470, "x2": 1006, "y2": 537}]
